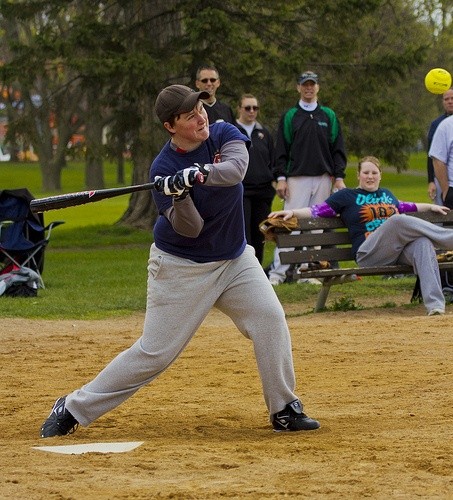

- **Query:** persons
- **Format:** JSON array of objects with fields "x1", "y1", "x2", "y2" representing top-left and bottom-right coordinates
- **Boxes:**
[
  {"x1": 40, "y1": 85, "x2": 319, "y2": 440},
  {"x1": 230, "y1": 93, "x2": 277, "y2": 266},
  {"x1": 425, "y1": 90, "x2": 453, "y2": 228},
  {"x1": 194, "y1": 65, "x2": 237, "y2": 127},
  {"x1": 427, "y1": 114, "x2": 453, "y2": 226},
  {"x1": 267, "y1": 156, "x2": 453, "y2": 316},
  {"x1": 267, "y1": 71, "x2": 348, "y2": 286}
]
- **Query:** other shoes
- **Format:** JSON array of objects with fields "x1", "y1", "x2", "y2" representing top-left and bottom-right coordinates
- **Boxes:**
[
  {"x1": 298, "y1": 278, "x2": 324, "y2": 285},
  {"x1": 427, "y1": 308, "x2": 445, "y2": 316},
  {"x1": 269, "y1": 279, "x2": 282, "y2": 286}
]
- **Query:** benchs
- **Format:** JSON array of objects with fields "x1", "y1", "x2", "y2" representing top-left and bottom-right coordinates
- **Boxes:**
[{"x1": 270, "y1": 209, "x2": 453, "y2": 313}]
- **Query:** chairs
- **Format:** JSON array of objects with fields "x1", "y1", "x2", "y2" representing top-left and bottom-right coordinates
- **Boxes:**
[{"x1": 0, "y1": 188, "x2": 65, "y2": 297}]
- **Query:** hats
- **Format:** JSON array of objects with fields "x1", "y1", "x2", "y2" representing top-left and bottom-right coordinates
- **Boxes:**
[
  {"x1": 154, "y1": 85, "x2": 210, "y2": 124},
  {"x1": 297, "y1": 70, "x2": 319, "y2": 85}
]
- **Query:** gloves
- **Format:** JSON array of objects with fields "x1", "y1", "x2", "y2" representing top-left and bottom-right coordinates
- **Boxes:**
[
  {"x1": 153, "y1": 175, "x2": 191, "y2": 202},
  {"x1": 173, "y1": 163, "x2": 210, "y2": 192}
]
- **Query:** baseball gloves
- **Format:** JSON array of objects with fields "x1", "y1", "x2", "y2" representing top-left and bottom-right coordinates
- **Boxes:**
[{"x1": 259, "y1": 217, "x2": 298, "y2": 242}]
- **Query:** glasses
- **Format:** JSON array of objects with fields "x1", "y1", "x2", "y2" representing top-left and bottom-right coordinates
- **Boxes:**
[
  {"x1": 241, "y1": 105, "x2": 261, "y2": 112},
  {"x1": 198, "y1": 76, "x2": 220, "y2": 83}
]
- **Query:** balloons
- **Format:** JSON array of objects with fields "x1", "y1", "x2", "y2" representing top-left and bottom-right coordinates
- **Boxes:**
[{"x1": 424, "y1": 68, "x2": 452, "y2": 94}]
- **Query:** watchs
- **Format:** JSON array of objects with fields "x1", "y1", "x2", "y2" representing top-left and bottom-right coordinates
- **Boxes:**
[{"x1": 199, "y1": 164, "x2": 209, "y2": 183}]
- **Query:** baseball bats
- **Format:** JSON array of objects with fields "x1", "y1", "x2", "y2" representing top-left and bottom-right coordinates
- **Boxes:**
[{"x1": 29, "y1": 172, "x2": 204, "y2": 213}]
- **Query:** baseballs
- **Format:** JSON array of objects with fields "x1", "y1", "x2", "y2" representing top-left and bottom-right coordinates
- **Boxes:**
[{"x1": 424, "y1": 68, "x2": 453, "y2": 94}]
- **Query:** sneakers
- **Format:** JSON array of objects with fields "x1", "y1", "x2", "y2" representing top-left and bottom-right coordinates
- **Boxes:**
[
  {"x1": 41, "y1": 395, "x2": 79, "y2": 440},
  {"x1": 272, "y1": 398, "x2": 320, "y2": 433}
]
